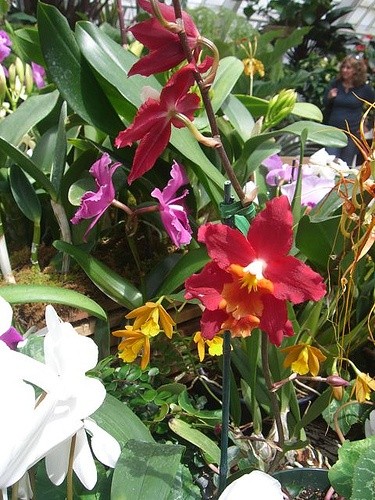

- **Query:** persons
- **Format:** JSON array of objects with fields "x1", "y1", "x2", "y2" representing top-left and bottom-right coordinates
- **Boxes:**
[{"x1": 322, "y1": 54, "x2": 375, "y2": 176}]
